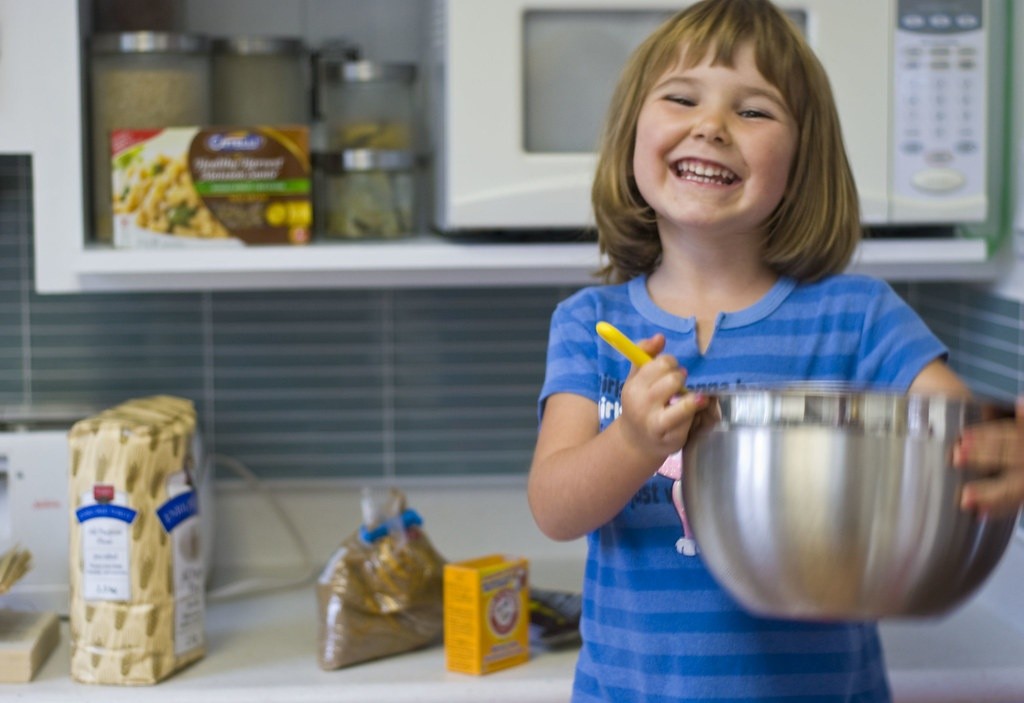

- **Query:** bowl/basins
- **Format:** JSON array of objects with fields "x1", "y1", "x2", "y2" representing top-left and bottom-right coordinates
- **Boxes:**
[{"x1": 683, "y1": 382, "x2": 1017, "y2": 621}]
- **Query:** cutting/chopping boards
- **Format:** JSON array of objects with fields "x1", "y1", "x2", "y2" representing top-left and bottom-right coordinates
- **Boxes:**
[{"x1": 0, "y1": 611, "x2": 61, "y2": 682}]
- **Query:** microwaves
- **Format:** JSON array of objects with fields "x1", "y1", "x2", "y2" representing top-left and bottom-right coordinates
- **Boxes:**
[{"x1": 415, "y1": 1, "x2": 991, "y2": 236}]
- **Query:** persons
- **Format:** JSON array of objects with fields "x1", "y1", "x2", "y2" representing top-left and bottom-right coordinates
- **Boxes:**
[{"x1": 524, "y1": 1, "x2": 1024, "y2": 702}]
